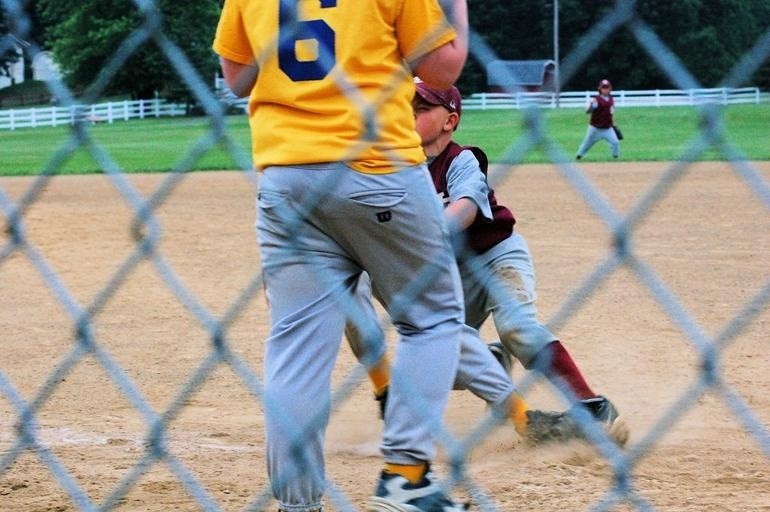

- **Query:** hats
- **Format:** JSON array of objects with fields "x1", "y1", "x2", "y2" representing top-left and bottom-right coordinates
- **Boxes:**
[
  {"x1": 597, "y1": 79, "x2": 613, "y2": 90},
  {"x1": 410, "y1": 73, "x2": 461, "y2": 130}
]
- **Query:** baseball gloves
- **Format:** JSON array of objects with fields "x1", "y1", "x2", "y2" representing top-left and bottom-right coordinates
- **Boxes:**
[{"x1": 613, "y1": 125, "x2": 623, "y2": 140}]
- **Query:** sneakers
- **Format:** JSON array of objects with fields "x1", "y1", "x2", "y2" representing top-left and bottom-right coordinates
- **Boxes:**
[
  {"x1": 374, "y1": 386, "x2": 388, "y2": 420},
  {"x1": 366, "y1": 464, "x2": 469, "y2": 511},
  {"x1": 486, "y1": 338, "x2": 516, "y2": 423},
  {"x1": 516, "y1": 395, "x2": 622, "y2": 443}
]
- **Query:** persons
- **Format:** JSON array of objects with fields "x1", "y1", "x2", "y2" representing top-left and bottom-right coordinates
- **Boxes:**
[
  {"x1": 411, "y1": 81, "x2": 621, "y2": 441},
  {"x1": 263, "y1": 266, "x2": 577, "y2": 447},
  {"x1": 575, "y1": 80, "x2": 624, "y2": 159},
  {"x1": 211, "y1": 0, "x2": 470, "y2": 512}
]
